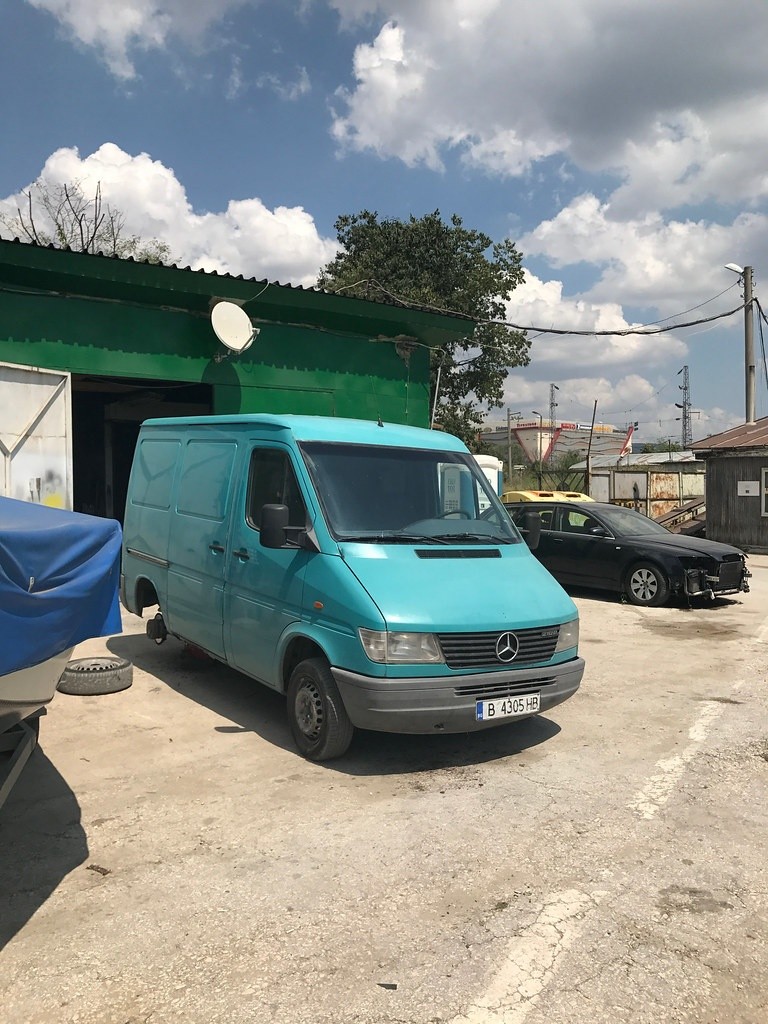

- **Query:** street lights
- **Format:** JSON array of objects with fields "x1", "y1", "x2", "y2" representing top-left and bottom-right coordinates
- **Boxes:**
[
  {"x1": 532, "y1": 411, "x2": 542, "y2": 492},
  {"x1": 725, "y1": 262, "x2": 755, "y2": 422}
]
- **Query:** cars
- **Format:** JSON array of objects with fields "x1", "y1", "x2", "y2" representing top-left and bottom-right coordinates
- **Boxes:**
[{"x1": 480, "y1": 501, "x2": 752, "y2": 606}]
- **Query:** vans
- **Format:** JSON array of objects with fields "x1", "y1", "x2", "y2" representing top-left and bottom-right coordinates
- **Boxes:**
[{"x1": 120, "y1": 412, "x2": 585, "y2": 761}]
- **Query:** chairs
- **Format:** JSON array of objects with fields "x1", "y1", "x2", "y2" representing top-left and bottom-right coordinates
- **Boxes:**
[
  {"x1": 369, "y1": 448, "x2": 419, "y2": 530},
  {"x1": 540, "y1": 512, "x2": 552, "y2": 529},
  {"x1": 583, "y1": 518, "x2": 599, "y2": 535}
]
